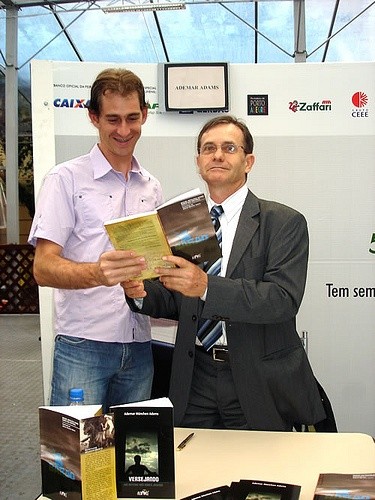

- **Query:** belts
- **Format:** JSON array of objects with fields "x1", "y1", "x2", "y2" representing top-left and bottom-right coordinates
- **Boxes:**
[{"x1": 196, "y1": 344, "x2": 229, "y2": 362}]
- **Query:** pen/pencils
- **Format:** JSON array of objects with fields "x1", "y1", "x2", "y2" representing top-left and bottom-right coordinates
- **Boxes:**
[{"x1": 176, "y1": 433, "x2": 194, "y2": 451}]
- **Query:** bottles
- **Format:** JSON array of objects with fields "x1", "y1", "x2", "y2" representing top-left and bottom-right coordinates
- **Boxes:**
[{"x1": 69, "y1": 388, "x2": 85, "y2": 406}]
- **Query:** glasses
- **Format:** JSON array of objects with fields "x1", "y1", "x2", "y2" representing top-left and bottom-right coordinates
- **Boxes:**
[{"x1": 197, "y1": 143, "x2": 247, "y2": 154}]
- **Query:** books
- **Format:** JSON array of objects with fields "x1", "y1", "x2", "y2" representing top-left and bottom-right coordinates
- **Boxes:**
[
  {"x1": 102, "y1": 188, "x2": 221, "y2": 287},
  {"x1": 40, "y1": 397, "x2": 301, "y2": 500}
]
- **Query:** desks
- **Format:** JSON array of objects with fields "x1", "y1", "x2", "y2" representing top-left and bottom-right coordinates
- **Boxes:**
[{"x1": 172, "y1": 427, "x2": 374, "y2": 500}]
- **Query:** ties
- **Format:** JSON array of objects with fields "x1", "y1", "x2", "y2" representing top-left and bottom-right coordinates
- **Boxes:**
[{"x1": 197, "y1": 205, "x2": 224, "y2": 353}]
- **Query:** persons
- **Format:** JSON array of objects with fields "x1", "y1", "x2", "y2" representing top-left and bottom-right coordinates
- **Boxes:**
[
  {"x1": 27, "y1": 68, "x2": 165, "y2": 414},
  {"x1": 120, "y1": 116, "x2": 327, "y2": 432}
]
